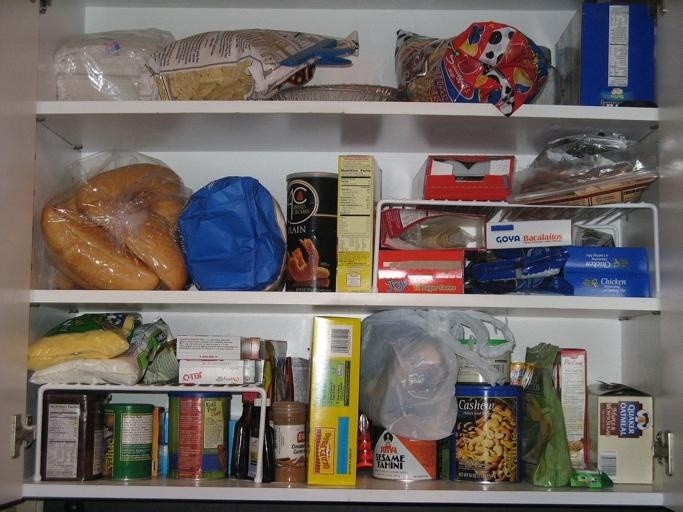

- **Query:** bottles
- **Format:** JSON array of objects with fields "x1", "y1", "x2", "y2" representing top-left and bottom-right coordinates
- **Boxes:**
[
  {"x1": 273, "y1": 401, "x2": 307, "y2": 484},
  {"x1": 231, "y1": 392, "x2": 276, "y2": 483}
]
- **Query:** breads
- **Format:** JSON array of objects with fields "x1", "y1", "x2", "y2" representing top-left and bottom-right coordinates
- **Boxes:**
[{"x1": 40, "y1": 163, "x2": 189, "y2": 290}]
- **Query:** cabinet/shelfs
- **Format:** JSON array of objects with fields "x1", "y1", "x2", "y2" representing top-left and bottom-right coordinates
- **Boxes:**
[{"x1": 0, "y1": 0, "x2": 683, "y2": 512}]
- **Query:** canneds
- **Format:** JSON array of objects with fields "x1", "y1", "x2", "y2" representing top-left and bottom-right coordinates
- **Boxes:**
[
  {"x1": 449, "y1": 382, "x2": 524, "y2": 483},
  {"x1": 286, "y1": 173, "x2": 337, "y2": 291},
  {"x1": 42, "y1": 394, "x2": 109, "y2": 481},
  {"x1": 167, "y1": 391, "x2": 231, "y2": 479},
  {"x1": 99, "y1": 403, "x2": 155, "y2": 481},
  {"x1": 370, "y1": 424, "x2": 439, "y2": 482}
]
